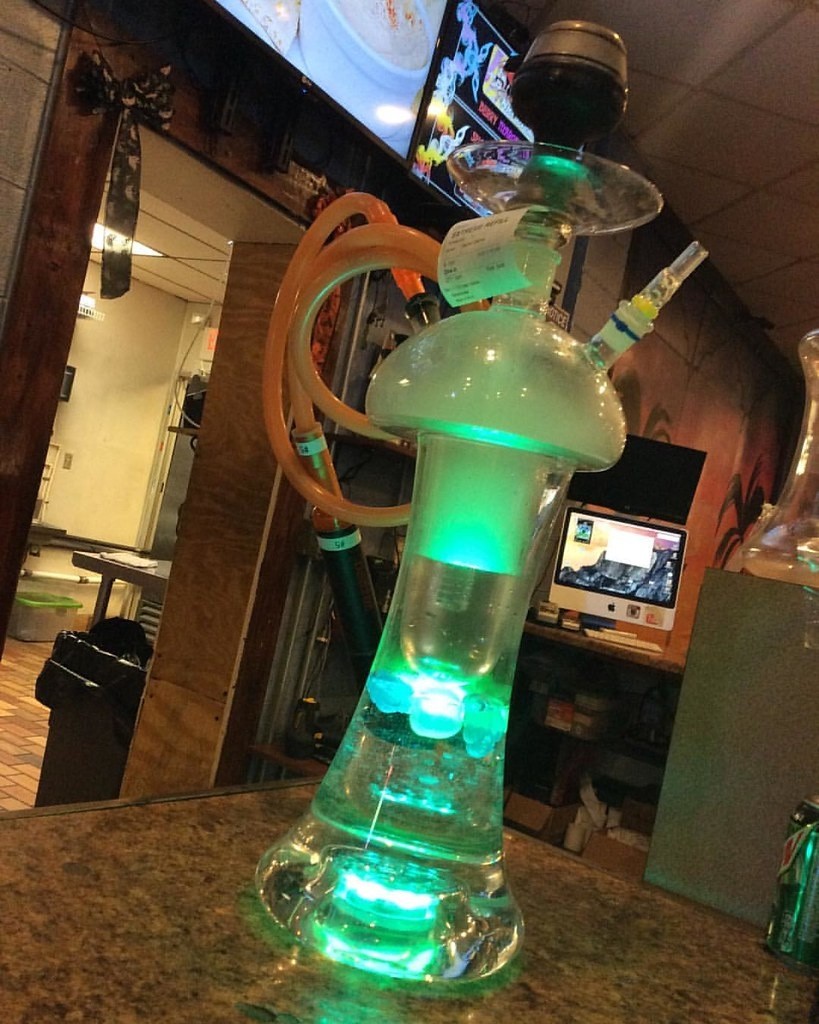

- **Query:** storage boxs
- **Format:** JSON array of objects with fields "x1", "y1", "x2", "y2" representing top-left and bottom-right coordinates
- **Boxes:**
[
  {"x1": 496, "y1": 777, "x2": 659, "y2": 882},
  {"x1": 6, "y1": 592, "x2": 83, "y2": 643}
]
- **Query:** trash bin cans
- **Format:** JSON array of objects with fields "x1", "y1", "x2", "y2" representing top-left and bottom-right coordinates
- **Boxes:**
[{"x1": 34, "y1": 630, "x2": 153, "y2": 807}]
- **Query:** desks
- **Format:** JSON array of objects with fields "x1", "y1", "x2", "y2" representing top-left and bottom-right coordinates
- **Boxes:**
[
  {"x1": 306, "y1": 555, "x2": 684, "y2": 876},
  {"x1": 0, "y1": 785, "x2": 819, "y2": 1024},
  {"x1": 72, "y1": 551, "x2": 172, "y2": 636}
]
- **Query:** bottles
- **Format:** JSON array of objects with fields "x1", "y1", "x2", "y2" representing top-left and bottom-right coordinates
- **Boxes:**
[{"x1": 738, "y1": 328, "x2": 819, "y2": 588}]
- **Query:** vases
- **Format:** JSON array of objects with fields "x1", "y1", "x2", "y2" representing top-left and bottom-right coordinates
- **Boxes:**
[{"x1": 740, "y1": 328, "x2": 819, "y2": 587}]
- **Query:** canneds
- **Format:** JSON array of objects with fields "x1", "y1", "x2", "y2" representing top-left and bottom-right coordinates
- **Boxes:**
[{"x1": 760, "y1": 792, "x2": 819, "y2": 969}]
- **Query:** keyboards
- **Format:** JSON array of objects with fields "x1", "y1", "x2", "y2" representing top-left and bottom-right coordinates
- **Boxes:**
[{"x1": 582, "y1": 628, "x2": 663, "y2": 653}]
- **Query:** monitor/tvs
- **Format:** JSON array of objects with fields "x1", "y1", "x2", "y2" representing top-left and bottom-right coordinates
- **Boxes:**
[
  {"x1": 548, "y1": 507, "x2": 687, "y2": 638},
  {"x1": 566, "y1": 434, "x2": 707, "y2": 525},
  {"x1": 205, "y1": 0, "x2": 589, "y2": 217}
]
